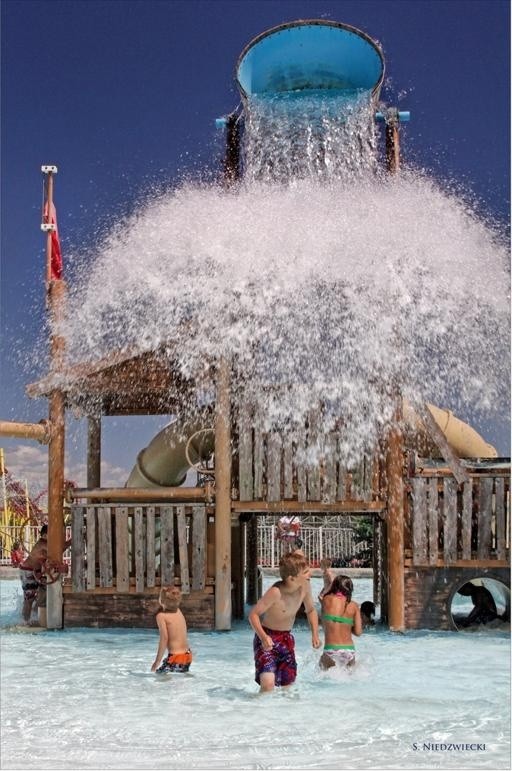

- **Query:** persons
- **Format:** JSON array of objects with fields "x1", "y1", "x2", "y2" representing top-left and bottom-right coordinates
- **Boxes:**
[
  {"x1": 457, "y1": 583, "x2": 497, "y2": 629},
  {"x1": 317, "y1": 576, "x2": 363, "y2": 672},
  {"x1": 318, "y1": 560, "x2": 334, "y2": 603},
  {"x1": 360, "y1": 602, "x2": 376, "y2": 628},
  {"x1": 151, "y1": 585, "x2": 193, "y2": 675},
  {"x1": 19, "y1": 525, "x2": 72, "y2": 628},
  {"x1": 11, "y1": 543, "x2": 23, "y2": 568},
  {"x1": 278, "y1": 516, "x2": 302, "y2": 553},
  {"x1": 293, "y1": 539, "x2": 304, "y2": 558},
  {"x1": 248, "y1": 553, "x2": 321, "y2": 696},
  {"x1": 296, "y1": 604, "x2": 307, "y2": 620}
]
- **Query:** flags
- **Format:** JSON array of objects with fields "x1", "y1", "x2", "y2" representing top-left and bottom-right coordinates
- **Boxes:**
[{"x1": 44, "y1": 200, "x2": 63, "y2": 282}]
